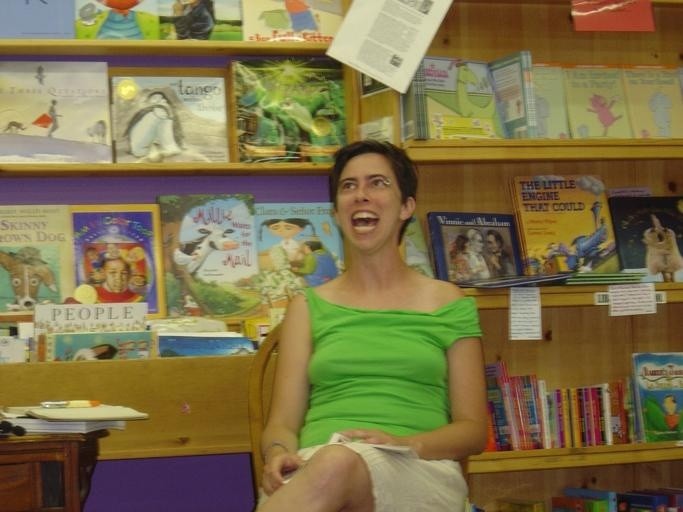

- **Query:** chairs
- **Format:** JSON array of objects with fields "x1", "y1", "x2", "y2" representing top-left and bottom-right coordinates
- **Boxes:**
[{"x1": 246, "y1": 318, "x2": 285, "y2": 490}]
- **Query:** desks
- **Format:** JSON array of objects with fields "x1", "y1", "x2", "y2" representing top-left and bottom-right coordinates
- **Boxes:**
[{"x1": 0, "y1": 431, "x2": 110, "y2": 511}]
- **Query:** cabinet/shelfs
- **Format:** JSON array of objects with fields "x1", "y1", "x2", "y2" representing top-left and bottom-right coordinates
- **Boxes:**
[{"x1": 0, "y1": 37, "x2": 682, "y2": 475}]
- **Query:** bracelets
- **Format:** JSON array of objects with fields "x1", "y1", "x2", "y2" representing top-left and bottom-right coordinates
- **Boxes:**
[{"x1": 262, "y1": 442, "x2": 289, "y2": 464}]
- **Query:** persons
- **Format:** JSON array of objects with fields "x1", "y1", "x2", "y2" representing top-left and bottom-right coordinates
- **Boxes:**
[{"x1": 251, "y1": 139, "x2": 491, "y2": 511}]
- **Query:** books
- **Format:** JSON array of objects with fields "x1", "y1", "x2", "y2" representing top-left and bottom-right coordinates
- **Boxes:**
[
  {"x1": 1, "y1": 0, "x2": 350, "y2": 42},
  {"x1": 2, "y1": 58, "x2": 349, "y2": 163},
  {"x1": 399, "y1": 172, "x2": 682, "y2": 290},
  {"x1": 481, "y1": 352, "x2": 682, "y2": 451},
  {"x1": 467, "y1": 484, "x2": 682, "y2": 512},
  {"x1": 354, "y1": 50, "x2": 683, "y2": 140},
  {"x1": 2, "y1": 193, "x2": 351, "y2": 362},
  {"x1": 0, "y1": 400, "x2": 151, "y2": 433}
]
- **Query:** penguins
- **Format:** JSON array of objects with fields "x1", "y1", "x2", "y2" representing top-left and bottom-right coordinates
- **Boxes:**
[{"x1": 124, "y1": 91, "x2": 188, "y2": 163}]
